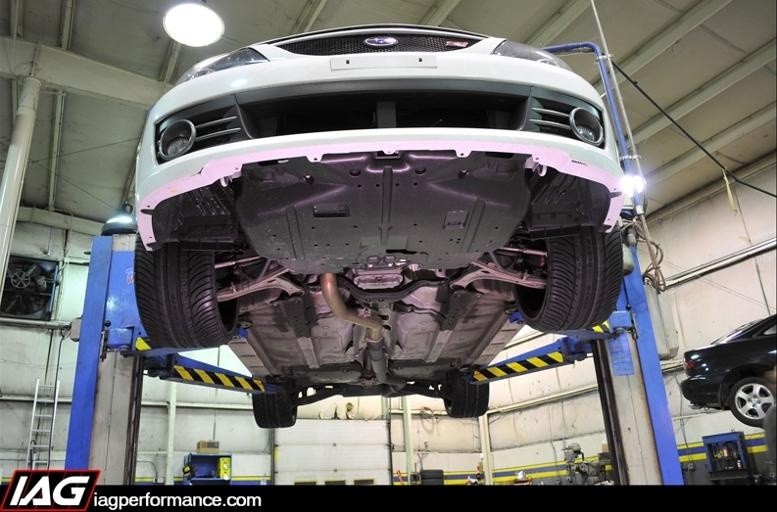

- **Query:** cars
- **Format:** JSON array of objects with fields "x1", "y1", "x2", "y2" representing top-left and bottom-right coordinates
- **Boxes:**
[
  {"x1": 677, "y1": 311, "x2": 777, "y2": 431},
  {"x1": 130, "y1": 24, "x2": 632, "y2": 425}
]
